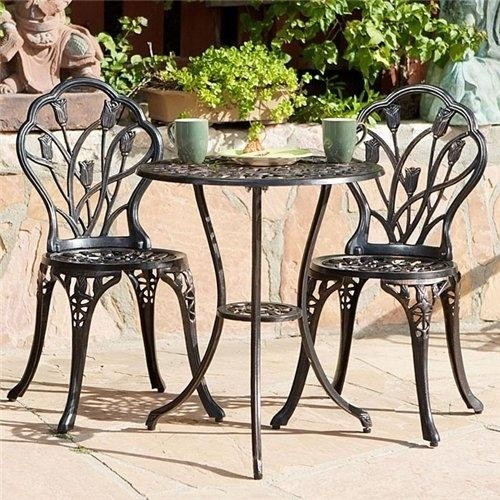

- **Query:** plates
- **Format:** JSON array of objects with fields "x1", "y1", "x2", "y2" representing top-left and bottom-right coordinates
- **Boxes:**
[{"x1": 220, "y1": 148, "x2": 313, "y2": 167}]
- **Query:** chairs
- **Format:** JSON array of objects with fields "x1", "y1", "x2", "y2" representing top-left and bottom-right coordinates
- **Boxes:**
[
  {"x1": 269, "y1": 83, "x2": 489, "y2": 448},
  {"x1": 7, "y1": 74, "x2": 226, "y2": 433}
]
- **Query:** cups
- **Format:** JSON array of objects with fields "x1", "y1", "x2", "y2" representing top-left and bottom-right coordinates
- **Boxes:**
[
  {"x1": 322, "y1": 117, "x2": 367, "y2": 164},
  {"x1": 167, "y1": 118, "x2": 209, "y2": 165}
]
